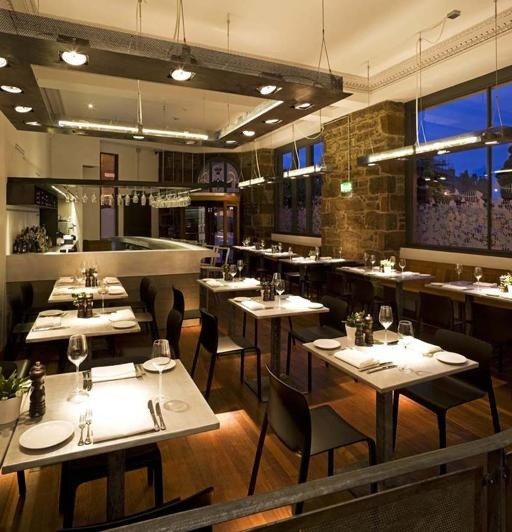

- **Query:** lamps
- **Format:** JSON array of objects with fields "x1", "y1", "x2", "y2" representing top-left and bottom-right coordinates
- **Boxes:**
[
  {"x1": 283, "y1": 109, "x2": 333, "y2": 180},
  {"x1": 357, "y1": 0, "x2": 512, "y2": 166},
  {"x1": 235, "y1": 132, "x2": 282, "y2": 190}
]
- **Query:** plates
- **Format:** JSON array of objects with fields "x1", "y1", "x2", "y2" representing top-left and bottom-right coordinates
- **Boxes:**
[
  {"x1": 312, "y1": 338, "x2": 469, "y2": 374},
  {"x1": 19, "y1": 266, "x2": 175, "y2": 453},
  {"x1": 233, "y1": 295, "x2": 326, "y2": 312}
]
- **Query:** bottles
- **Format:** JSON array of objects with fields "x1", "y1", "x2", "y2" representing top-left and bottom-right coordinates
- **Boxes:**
[
  {"x1": 354, "y1": 313, "x2": 373, "y2": 346},
  {"x1": 10, "y1": 223, "x2": 49, "y2": 255}
]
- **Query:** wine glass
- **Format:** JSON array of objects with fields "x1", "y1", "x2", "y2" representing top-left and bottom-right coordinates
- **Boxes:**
[
  {"x1": 219, "y1": 259, "x2": 248, "y2": 286},
  {"x1": 270, "y1": 269, "x2": 288, "y2": 311},
  {"x1": 46, "y1": 181, "x2": 194, "y2": 211},
  {"x1": 449, "y1": 260, "x2": 484, "y2": 295},
  {"x1": 350, "y1": 251, "x2": 430, "y2": 283},
  {"x1": 239, "y1": 236, "x2": 347, "y2": 264},
  {"x1": 379, "y1": 304, "x2": 413, "y2": 361}
]
